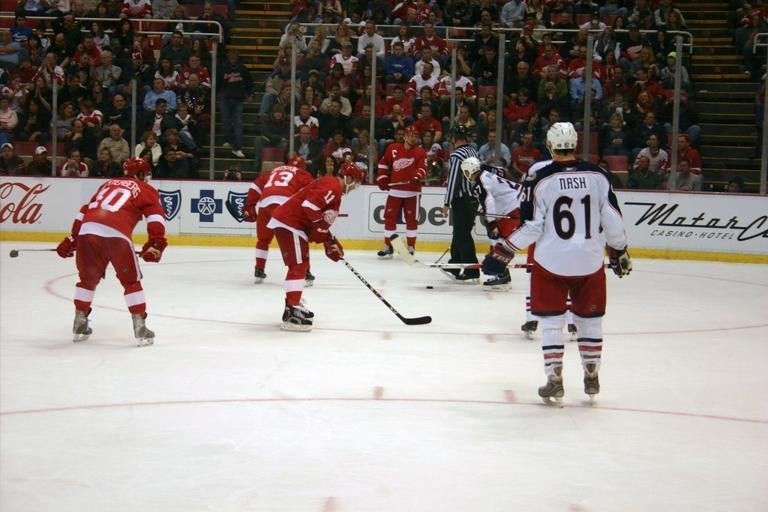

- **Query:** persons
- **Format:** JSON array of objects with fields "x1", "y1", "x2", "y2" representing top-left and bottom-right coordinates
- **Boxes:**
[
  {"x1": 266, "y1": 160, "x2": 364, "y2": 325},
  {"x1": 240, "y1": 153, "x2": 315, "y2": 288},
  {"x1": 375, "y1": 126, "x2": 428, "y2": 261},
  {"x1": 725, "y1": 176, "x2": 747, "y2": 192},
  {"x1": 254, "y1": 1, "x2": 705, "y2": 195},
  {"x1": 727, "y1": 1, "x2": 768, "y2": 122},
  {"x1": 0, "y1": 1, "x2": 231, "y2": 180},
  {"x1": 435, "y1": 124, "x2": 484, "y2": 281},
  {"x1": 55, "y1": 157, "x2": 166, "y2": 338},
  {"x1": 520, "y1": 241, "x2": 577, "y2": 343},
  {"x1": 458, "y1": 155, "x2": 526, "y2": 295},
  {"x1": 480, "y1": 122, "x2": 633, "y2": 399},
  {"x1": 215, "y1": 49, "x2": 253, "y2": 158}
]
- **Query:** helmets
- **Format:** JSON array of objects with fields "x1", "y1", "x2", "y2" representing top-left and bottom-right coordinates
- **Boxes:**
[
  {"x1": 338, "y1": 159, "x2": 362, "y2": 200},
  {"x1": 122, "y1": 155, "x2": 150, "y2": 176},
  {"x1": 544, "y1": 122, "x2": 577, "y2": 152},
  {"x1": 447, "y1": 124, "x2": 465, "y2": 144},
  {"x1": 460, "y1": 157, "x2": 481, "y2": 184},
  {"x1": 287, "y1": 156, "x2": 306, "y2": 170},
  {"x1": 402, "y1": 126, "x2": 418, "y2": 146}
]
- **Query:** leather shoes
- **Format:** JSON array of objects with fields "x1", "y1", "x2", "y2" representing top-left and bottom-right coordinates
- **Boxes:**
[
  {"x1": 443, "y1": 263, "x2": 460, "y2": 275},
  {"x1": 456, "y1": 273, "x2": 479, "y2": 280}
]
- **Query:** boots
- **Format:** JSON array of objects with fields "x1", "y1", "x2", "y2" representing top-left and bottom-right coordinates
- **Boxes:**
[
  {"x1": 131, "y1": 312, "x2": 154, "y2": 338},
  {"x1": 72, "y1": 307, "x2": 93, "y2": 334}
]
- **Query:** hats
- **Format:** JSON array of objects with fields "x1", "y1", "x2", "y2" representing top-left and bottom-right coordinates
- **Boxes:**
[
  {"x1": 34, "y1": 146, "x2": 47, "y2": 155},
  {"x1": 1, "y1": 143, "x2": 13, "y2": 149},
  {"x1": 308, "y1": 69, "x2": 320, "y2": 75},
  {"x1": 667, "y1": 51, "x2": 676, "y2": 58}
]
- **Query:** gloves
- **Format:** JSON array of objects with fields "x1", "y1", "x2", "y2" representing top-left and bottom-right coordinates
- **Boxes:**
[
  {"x1": 308, "y1": 218, "x2": 332, "y2": 243},
  {"x1": 480, "y1": 242, "x2": 513, "y2": 276},
  {"x1": 323, "y1": 236, "x2": 343, "y2": 262},
  {"x1": 605, "y1": 242, "x2": 632, "y2": 279},
  {"x1": 377, "y1": 176, "x2": 389, "y2": 191},
  {"x1": 56, "y1": 234, "x2": 76, "y2": 258},
  {"x1": 408, "y1": 176, "x2": 419, "y2": 184},
  {"x1": 243, "y1": 205, "x2": 256, "y2": 222},
  {"x1": 487, "y1": 221, "x2": 498, "y2": 240},
  {"x1": 139, "y1": 239, "x2": 168, "y2": 263}
]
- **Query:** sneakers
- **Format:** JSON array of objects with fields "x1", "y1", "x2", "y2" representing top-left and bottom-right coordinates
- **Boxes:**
[
  {"x1": 407, "y1": 245, "x2": 414, "y2": 255},
  {"x1": 299, "y1": 302, "x2": 313, "y2": 318},
  {"x1": 282, "y1": 298, "x2": 311, "y2": 324},
  {"x1": 583, "y1": 377, "x2": 599, "y2": 394},
  {"x1": 377, "y1": 245, "x2": 392, "y2": 256},
  {"x1": 482, "y1": 268, "x2": 576, "y2": 333},
  {"x1": 305, "y1": 271, "x2": 314, "y2": 280},
  {"x1": 223, "y1": 141, "x2": 244, "y2": 157},
  {"x1": 254, "y1": 266, "x2": 265, "y2": 278},
  {"x1": 537, "y1": 376, "x2": 564, "y2": 397}
]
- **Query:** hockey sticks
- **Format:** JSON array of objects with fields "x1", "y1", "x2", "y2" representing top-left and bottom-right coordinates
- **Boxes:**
[
  {"x1": 414, "y1": 256, "x2": 610, "y2": 270},
  {"x1": 339, "y1": 254, "x2": 434, "y2": 324},
  {"x1": 11, "y1": 250, "x2": 146, "y2": 262},
  {"x1": 386, "y1": 177, "x2": 439, "y2": 187}
]
